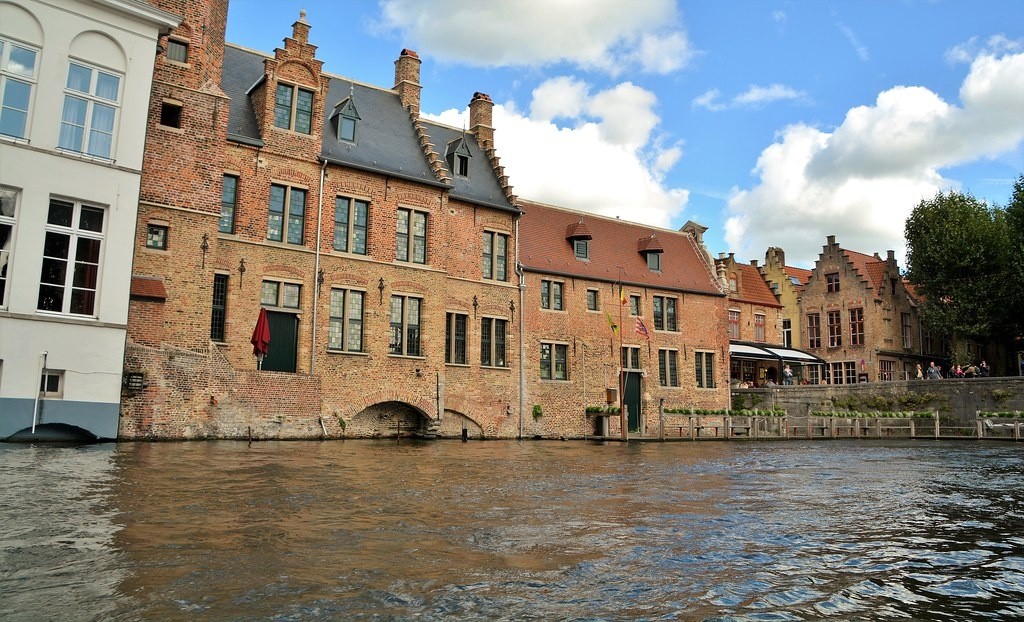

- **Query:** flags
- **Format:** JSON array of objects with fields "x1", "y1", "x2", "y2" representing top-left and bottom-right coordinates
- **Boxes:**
[
  {"x1": 635, "y1": 317, "x2": 651, "y2": 339},
  {"x1": 619, "y1": 279, "x2": 627, "y2": 306},
  {"x1": 605, "y1": 310, "x2": 620, "y2": 337}
]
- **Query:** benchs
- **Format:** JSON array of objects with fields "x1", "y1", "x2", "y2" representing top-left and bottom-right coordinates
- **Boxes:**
[{"x1": 947, "y1": 373, "x2": 982, "y2": 378}]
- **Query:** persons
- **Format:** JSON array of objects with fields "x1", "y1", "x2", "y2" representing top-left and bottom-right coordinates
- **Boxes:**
[
  {"x1": 730, "y1": 365, "x2": 828, "y2": 388},
  {"x1": 913, "y1": 360, "x2": 992, "y2": 380}
]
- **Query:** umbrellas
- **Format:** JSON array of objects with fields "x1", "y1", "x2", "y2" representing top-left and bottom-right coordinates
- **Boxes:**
[{"x1": 250, "y1": 308, "x2": 270, "y2": 370}]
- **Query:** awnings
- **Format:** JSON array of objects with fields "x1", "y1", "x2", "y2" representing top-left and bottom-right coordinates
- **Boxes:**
[{"x1": 728, "y1": 340, "x2": 826, "y2": 366}]
